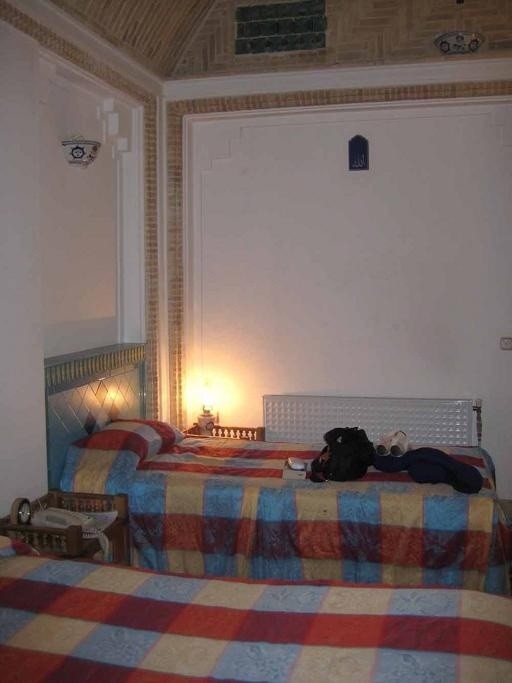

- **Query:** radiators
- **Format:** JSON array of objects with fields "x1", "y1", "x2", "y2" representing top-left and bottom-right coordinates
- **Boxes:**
[{"x1": 263, "y1": 395, "x2": 473, "y2": 446}]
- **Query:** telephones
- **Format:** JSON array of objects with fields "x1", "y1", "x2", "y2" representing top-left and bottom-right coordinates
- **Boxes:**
[{"x1": 31, "y1": 508, "x2": 94, "y2": 530}]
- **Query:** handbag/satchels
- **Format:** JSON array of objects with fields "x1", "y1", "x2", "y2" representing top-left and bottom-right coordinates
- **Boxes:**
[{"x1": 311, "y1": 427, "x2": 374, "y2": 481}]
